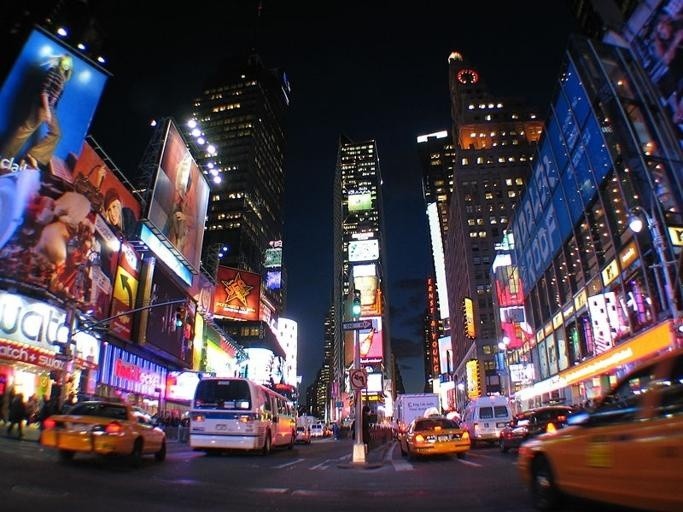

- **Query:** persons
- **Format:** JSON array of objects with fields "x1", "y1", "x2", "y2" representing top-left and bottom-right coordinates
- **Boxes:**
[
  {"x1": 0, "y1": 154, "x2": 138, "y2": 322},
  {"x1": 0, "y1": 52, "x2": 73, "y2": 173},
  {"x1": 329, "y1": 405, "x2": 379, "y2": 455},
  {"x1": 170, "y1": 168, "x2": 196, "y2": 254},
  {"x1": 146, "y1": 288, "x2": 192, "y2": 363},
  {"x1": 147, "y1": 407, "x2": 190, "y2": 444},
  {"x1": 0, "y1": 386, "x2": 74, "y2": 443}
]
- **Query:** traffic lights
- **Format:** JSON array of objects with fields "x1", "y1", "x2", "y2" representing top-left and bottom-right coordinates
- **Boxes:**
[
  {"x1": 174, "y1": 314, "x2": 185, "y2": 338},
  {"x1": 352, "y1": 291, "x2": 363, "y2": 320}
]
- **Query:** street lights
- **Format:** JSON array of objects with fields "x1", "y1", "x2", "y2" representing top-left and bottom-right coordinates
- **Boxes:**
[{"x1": 627, "y1": 205, "x2": 678, "y2": 319}]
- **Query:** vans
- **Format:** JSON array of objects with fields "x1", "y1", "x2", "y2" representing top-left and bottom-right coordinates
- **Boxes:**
[{"x1": 463, "y1": 397, "x2": 513, "y2": 447}]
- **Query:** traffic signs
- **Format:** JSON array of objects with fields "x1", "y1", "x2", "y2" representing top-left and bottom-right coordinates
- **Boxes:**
[{"x1": 340, "y1": 320, "x2": 374, "y2": 332}]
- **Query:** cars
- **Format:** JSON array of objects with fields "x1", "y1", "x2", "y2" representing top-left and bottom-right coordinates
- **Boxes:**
[
  {"x1": 36, "y1": 402, "x2": 166, "y2": 469},
  {"x1": 298, "y1": 413, "x2": 326, "y2": 445},
  {"x1": 397, "y1": 418, "x2": 473, "y2": 457},
  {"x1": 501, "y1": 369, "x2": 683, "y2": 512}
]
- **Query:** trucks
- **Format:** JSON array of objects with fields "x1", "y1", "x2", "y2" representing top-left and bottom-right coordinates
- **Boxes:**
[{"x1": 395, "y1": 394, "x2": 441, "y2": 433}]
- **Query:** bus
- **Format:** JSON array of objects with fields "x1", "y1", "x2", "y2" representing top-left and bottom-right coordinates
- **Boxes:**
[{"x1": 187, "y1": 375, "x2": 298, "y2": 452}]
